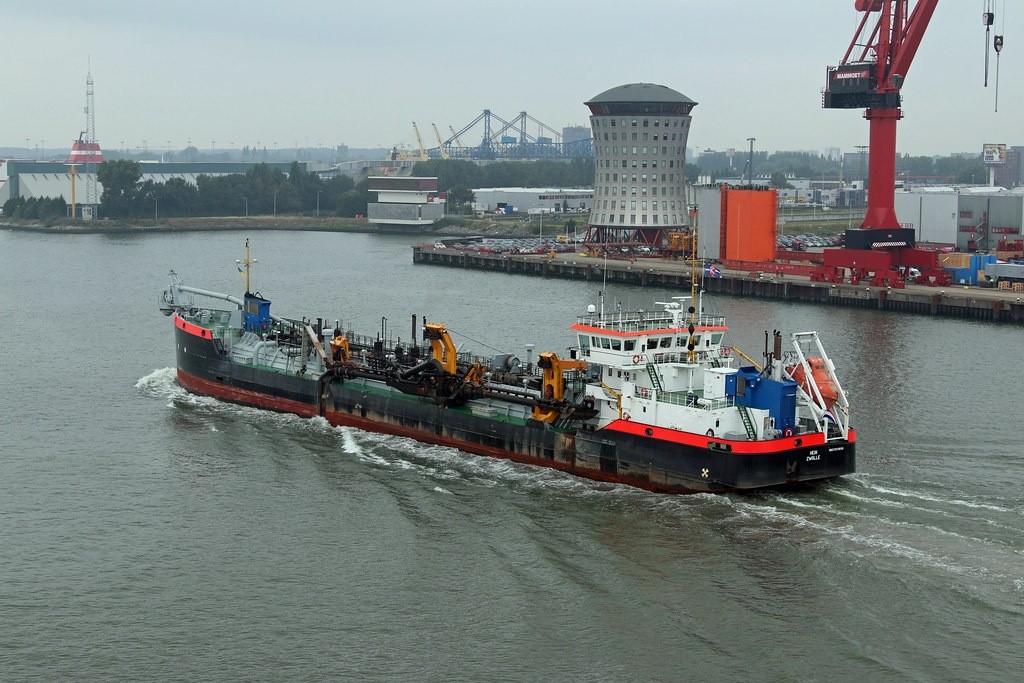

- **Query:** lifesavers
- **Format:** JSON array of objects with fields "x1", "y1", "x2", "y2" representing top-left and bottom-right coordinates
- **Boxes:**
[
  {"x1": 623, "y1": 412, "x2": 629, "y2": 420},
  {"x1": 721, "y1": 348, "x2": 731, "y2": 355},
  {"x1": 641, "y1": 388, "x2": 648, "y2": 398},
  {"x1": 633, "y1": 355, "x2": 639, "y2": 363}
]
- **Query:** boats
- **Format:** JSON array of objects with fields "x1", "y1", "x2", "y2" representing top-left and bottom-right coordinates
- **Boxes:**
[{"x1": 159, "y1": 206, "x2": 857, "y2": 496}]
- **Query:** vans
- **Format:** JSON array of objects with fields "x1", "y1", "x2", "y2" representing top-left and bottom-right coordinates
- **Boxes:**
[{"x1": 899, "y1": 267, "x2": 921, "y2": 281}]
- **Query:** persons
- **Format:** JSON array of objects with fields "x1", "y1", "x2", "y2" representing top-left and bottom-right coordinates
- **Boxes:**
[
  {"x1": 780, "y1": 268, "x2": 784, "y2": 277},
  {"x1": 607, "y1": 344, "x2": 610, "y2": 349},
  {"x1": 776, "y1": 268, "x2": 778, "y2": 277},
  {"x1": 648, "y1": 339, "x2": 657, "y2": 348},
  {"x1": 625, "y1": 341, "x2": 634, "y2": 350}
]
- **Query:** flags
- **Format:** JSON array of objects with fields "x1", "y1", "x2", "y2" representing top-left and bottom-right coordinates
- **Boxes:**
[{"x1": 704, "y1": 262, "x2": 721, "y2": 278}]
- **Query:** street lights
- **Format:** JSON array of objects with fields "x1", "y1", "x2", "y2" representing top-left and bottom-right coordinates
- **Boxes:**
[
  {"x1": 151, "y1": 197, "x2": 159, "y2": 219},
  {"x1": 316, "y1": 189, "x2": 323, "y2": 217},
  {"x1": 272, "y1": 188, "x2": 281, "y2": 216},
  {"x1": 242, "y1": 196, "x2": 250, "y2": 216}
]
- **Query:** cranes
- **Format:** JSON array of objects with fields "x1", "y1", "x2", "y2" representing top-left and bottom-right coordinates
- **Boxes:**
[{"x1": 820, "y1": 0, "x2": 1006, "y2": 289}]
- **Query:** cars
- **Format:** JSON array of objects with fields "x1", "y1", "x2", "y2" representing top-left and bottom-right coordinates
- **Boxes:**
[
  {"x1": 776, "y1": 232, "x2": 845, "y2": 248},
  {"x1": 568, "y1": 236, "x2": 588, "y2": 243},
  {"x1": 453, "y1": 242, "x2": 463, "y2": 249},
  {"x1": 467, "y1": 237, "x2": 567, "y2": 255},
  {"x1": 621, "y1": 246, "x2": 651, "y2": 253},
  {"x1": 434, "y1": 242, "x2": 446, "y2": 249}
]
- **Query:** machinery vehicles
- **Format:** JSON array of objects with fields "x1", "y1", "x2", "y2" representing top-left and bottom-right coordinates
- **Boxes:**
[
  {"x1": 448, "y1": 125, "x2": 472, "y2": 161},
  {"x1": 431, "y1": 122, "x2": 450, "y2": 160},
  {"x1": 384, "y1": 121, "x2": 428, "y2": 160}
]
